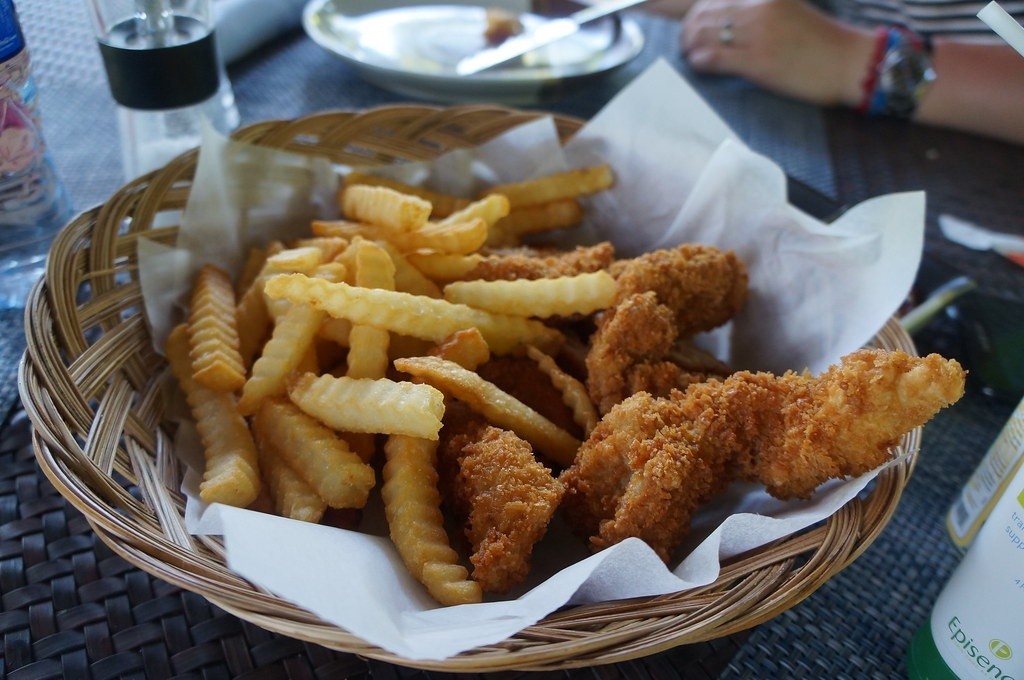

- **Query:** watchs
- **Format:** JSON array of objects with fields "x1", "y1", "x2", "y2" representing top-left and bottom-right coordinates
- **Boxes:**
[{"x1": 853, "y1": 23, "x2": 939, "y2": 123}]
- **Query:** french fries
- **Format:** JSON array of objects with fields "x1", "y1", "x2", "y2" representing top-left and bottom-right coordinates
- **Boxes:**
[{"x1": 166, "y1": 165, "x2": 616, "y2": 604}]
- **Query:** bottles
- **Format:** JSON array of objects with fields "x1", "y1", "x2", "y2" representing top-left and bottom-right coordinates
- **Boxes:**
[
  {"x1": 85, "y1": 0, "x2": 244, "y2": 234},
  {"x1": 0, "y1": 0, "x2": 78, "y2": 311}
]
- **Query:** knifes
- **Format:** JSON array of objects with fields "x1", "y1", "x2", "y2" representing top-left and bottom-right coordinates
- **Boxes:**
[{"x1": 458, "y1": 0, "x2": 648, "y2": 78}]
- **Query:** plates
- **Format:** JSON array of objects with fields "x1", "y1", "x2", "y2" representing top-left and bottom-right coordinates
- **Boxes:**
[{"x1": 302, "y1": 0, "x2": 643, "y2": 110}]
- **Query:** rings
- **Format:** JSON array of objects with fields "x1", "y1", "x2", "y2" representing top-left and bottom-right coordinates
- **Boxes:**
[{"x1": 719, "y1": 23, "x2": 733, "y2": 46}]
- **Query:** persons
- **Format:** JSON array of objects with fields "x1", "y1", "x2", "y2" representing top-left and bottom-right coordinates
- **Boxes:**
[{"x1": 638, "y1": 0, "x2": 1024, "y2": 144}]
